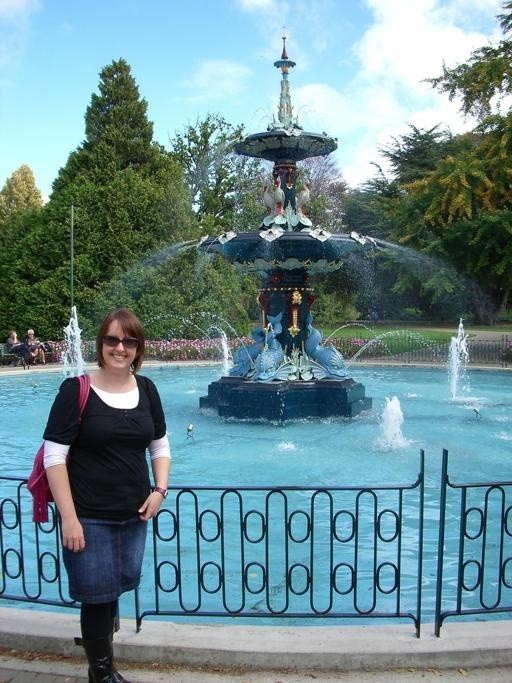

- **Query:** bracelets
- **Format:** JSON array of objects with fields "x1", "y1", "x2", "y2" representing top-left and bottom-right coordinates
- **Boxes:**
[{"x1": 152, "y1": 486, "x2": 168, "y2": 498}]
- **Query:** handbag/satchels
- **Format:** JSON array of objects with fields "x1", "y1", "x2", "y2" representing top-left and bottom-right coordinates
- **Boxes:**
[{"x1": 27, "y1": 441, "x2": 71, "y2": 502}]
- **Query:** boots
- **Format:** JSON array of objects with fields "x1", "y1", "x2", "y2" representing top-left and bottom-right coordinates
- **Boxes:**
[{"x1": 82, "y1": 635, "x2": 130, "y2": 683}]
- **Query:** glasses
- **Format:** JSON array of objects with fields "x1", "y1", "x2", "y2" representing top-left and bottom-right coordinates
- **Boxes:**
[{"x1": 101, "y1": 335, "x2": 140, "y2": 349}]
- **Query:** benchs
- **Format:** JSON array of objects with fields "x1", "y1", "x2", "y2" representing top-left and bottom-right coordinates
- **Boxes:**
[{"x1": 0, "y1": 343, "x2": 53, "y2": 365}]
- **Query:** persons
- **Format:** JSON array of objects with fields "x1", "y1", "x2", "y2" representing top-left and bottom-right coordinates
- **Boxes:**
[
  {"x1": 25, "y1": 328, "x2": 47, "y2": 365},
  {"x1": 40, "y1": 307, "x2": 171, "y2": 682},
  {"x1": 6, "y1": 329, "x2": 37, "y2": 365}
]
